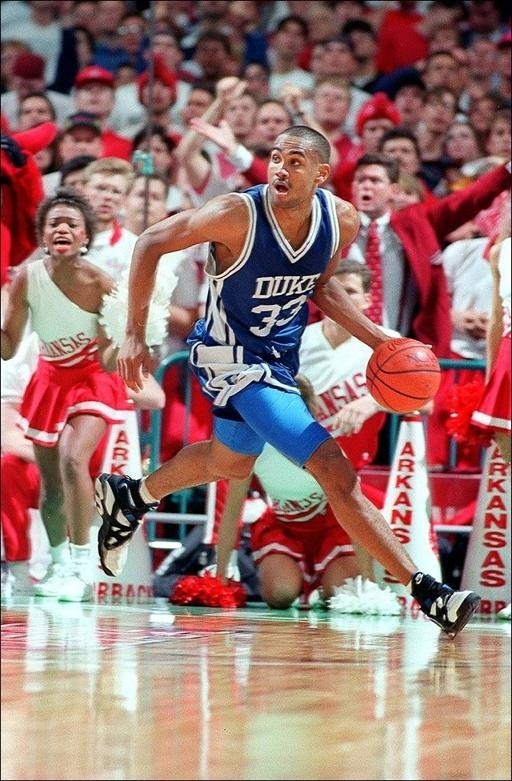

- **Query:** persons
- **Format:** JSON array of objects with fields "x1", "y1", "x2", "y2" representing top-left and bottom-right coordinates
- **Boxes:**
[
  {"x1": 1, "y1": 1, "x2": 511, "y2": 359},
  {"x1": 281, "y1": 259, "x2": 434, "y2": 481},
  {"x1": 1, "y1": 193, "x2": 164, "y2": 602},
  {"x1": 247, "y1": 377, "x2": 371, "y2": 608},
  {"x1": 1, "y1": 298, "x2": 47, "y2": 608},
  {"x1": 470, "y1": 194, "x2": 511, "y2": 620},
  {"x1": 92, "y1": 124, "x2": 481, "y2": 641}
]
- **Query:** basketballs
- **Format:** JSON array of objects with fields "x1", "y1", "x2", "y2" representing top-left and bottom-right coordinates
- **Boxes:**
[{"x1": 366, "y1": 338, "x2": 439, "y2": 411}]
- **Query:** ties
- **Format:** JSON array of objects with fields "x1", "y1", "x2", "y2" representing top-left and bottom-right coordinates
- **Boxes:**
[{"x1": 364, "y1": 224, "x2": 386, "y2": 328}]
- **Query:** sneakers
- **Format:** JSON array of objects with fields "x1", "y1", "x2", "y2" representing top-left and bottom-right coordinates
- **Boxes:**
[
  {"x1": 407, "y1": 570, "x2": 482, "y2": 641},
  {"x1": 94, "y1": 471, "x2": 159, "y2": 578},
  {"x1": 58, "y1": 569, "x2": 98, "y2": 603},
  {"x1": 33, "y1": 562, "x2": 70, "y2": 597}
]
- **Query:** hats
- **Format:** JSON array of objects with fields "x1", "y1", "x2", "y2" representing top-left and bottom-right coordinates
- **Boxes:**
[
  {"x1": 63, "y1": 112, "x2": 104, "y2": 136},
  {"x1": 73, "y1": 62, "x2": 118, "y2": 89},
  {"x1": 354, "y1": 91, "x2": 400, "y2": 141},
  {"x1": 12, "y1": 54, "x2": 46, "y2": 80},
  {"x1": 138, "y1": 57, "x2": 177, "y2": 105}
]
